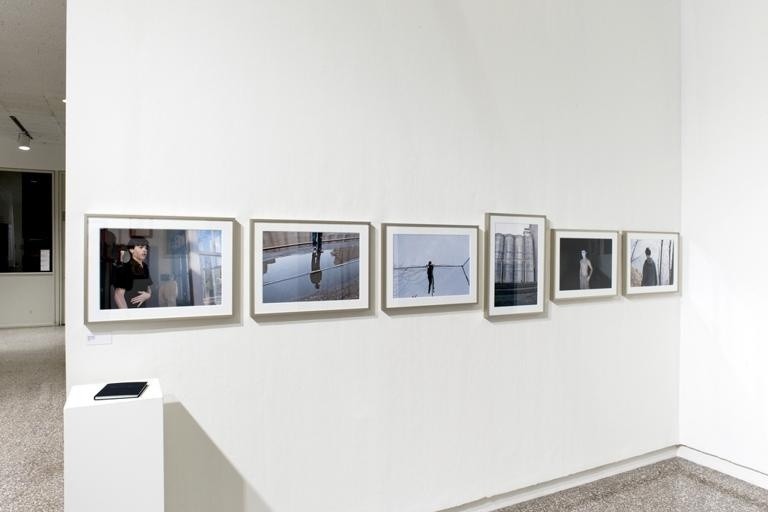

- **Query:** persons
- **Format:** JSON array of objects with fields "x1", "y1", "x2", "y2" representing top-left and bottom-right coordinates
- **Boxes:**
[
  {"x1": 312, "y1": 232, "x2": 324, "y2": 254},
  {"x1": 310, "y1": 253, "x2": 322, "y2": 289},
  {"x1": 113, "y1": 235, "x2": 153, "y2": 308},
  {"x1": 641, "y1": 248, "x2": 658, "y2": 286},
  {"x1": 426, "y1": 261, "x2": 435, "y2": 294},
  {"x1": 579, "y1": 248, "x2": 593, "y2": 289}
]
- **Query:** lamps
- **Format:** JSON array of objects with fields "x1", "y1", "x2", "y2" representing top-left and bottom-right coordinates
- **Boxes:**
[{"x1": 16, "y1": 131, "x2": 31, "y2": 151}]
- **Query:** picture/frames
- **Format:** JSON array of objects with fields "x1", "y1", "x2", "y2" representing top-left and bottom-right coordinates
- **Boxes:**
[
  {"x1": 380, "y1": 223, "x2": 479, "y2": 312},
  {"x1": 551, "y1": 229, "x2": 621, "y2": 303},
  {"x1": 622, "y1": 230, "x2": 681, "y2": 298},
  {"x1": 83, "y1": 213, "x2": 237, "y2": 326},
  {"x1": 248, "y1": 218, "x2": 372, "y2": 320},
  {"x1": 483, "y1": 212, "x2": 548, "y2": 320}
]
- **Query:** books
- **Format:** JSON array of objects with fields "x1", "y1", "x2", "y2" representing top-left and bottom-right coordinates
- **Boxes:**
[{"x1": 94, "y1": 382, "x2": 148, "y2": 400}]
